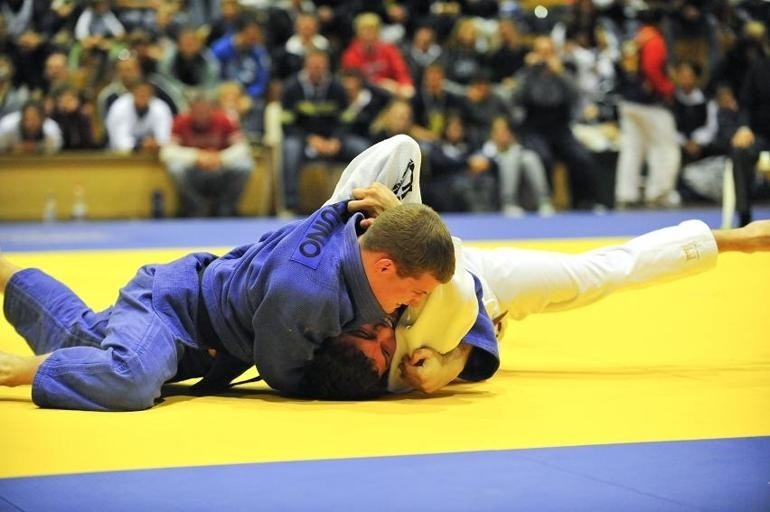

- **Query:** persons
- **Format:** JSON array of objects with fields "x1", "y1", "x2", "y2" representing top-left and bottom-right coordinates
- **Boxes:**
[
  {"x1": 1, "y1": 192, "x2": 501, "y2": 412},
  {"x1": 1, "y1": 1, "x2": 770, "y2": 227},
  {"x1": 295, "y1": 136, "x2": 770, "y2": 403}
]
